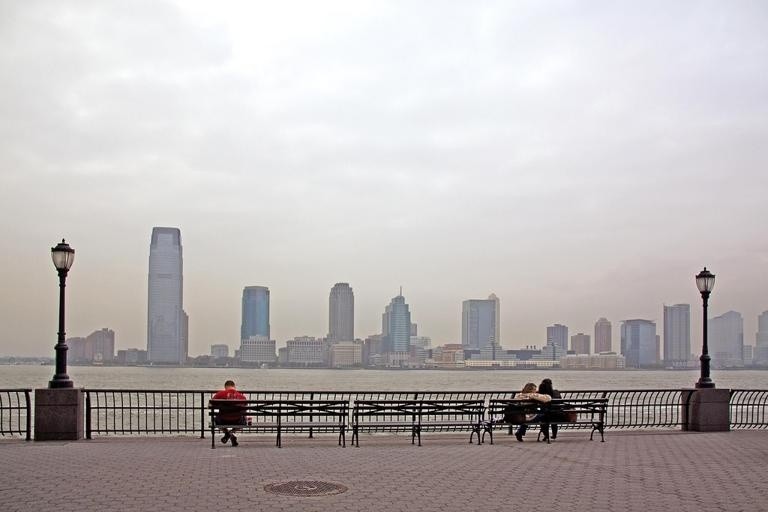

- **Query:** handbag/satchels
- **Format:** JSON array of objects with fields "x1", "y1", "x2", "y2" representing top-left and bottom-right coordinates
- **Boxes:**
[{"x1": 567, "y1": 408, "x2": 577, "y2": 421}]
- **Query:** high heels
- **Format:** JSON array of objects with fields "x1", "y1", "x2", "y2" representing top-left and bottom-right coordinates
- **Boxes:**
[{"x1": 543, "y1": 434, "x2": 556, "y2": 443}]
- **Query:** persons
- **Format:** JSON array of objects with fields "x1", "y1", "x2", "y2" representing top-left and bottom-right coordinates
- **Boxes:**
[
  {"x1": 212, "y1": 381, "x2": 247, "y2": 447},
  {"x1": 514, "y1": 379, "x2": 561, "y2": 442}
]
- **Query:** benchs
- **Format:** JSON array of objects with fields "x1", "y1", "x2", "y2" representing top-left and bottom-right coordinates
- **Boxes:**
[
  {"x1": 348, "y1": 400, "x2": 488, "y2": 447},
  {"x1": 480, "y1": 398, "x2": 610, "y2": 445},
  {"x1": 208, "y1": 398, "x2": 350, "y2": 449}
]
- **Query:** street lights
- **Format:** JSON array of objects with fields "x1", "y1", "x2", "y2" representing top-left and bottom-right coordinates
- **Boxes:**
[
  {"x1": 694, "y1": 265, "x2": 717, "y2": 387},
  {"x1": 49, "y1": 235, "x2": 76, "y2": 387}
]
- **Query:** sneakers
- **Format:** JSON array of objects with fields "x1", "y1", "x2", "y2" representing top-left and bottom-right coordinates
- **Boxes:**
[
  {"x1": 515, "y1": 432, "x2": 523, "y2": 442},
  {"x1": 221, "y1": 432, "x2": 238, "y2": 446}
]
